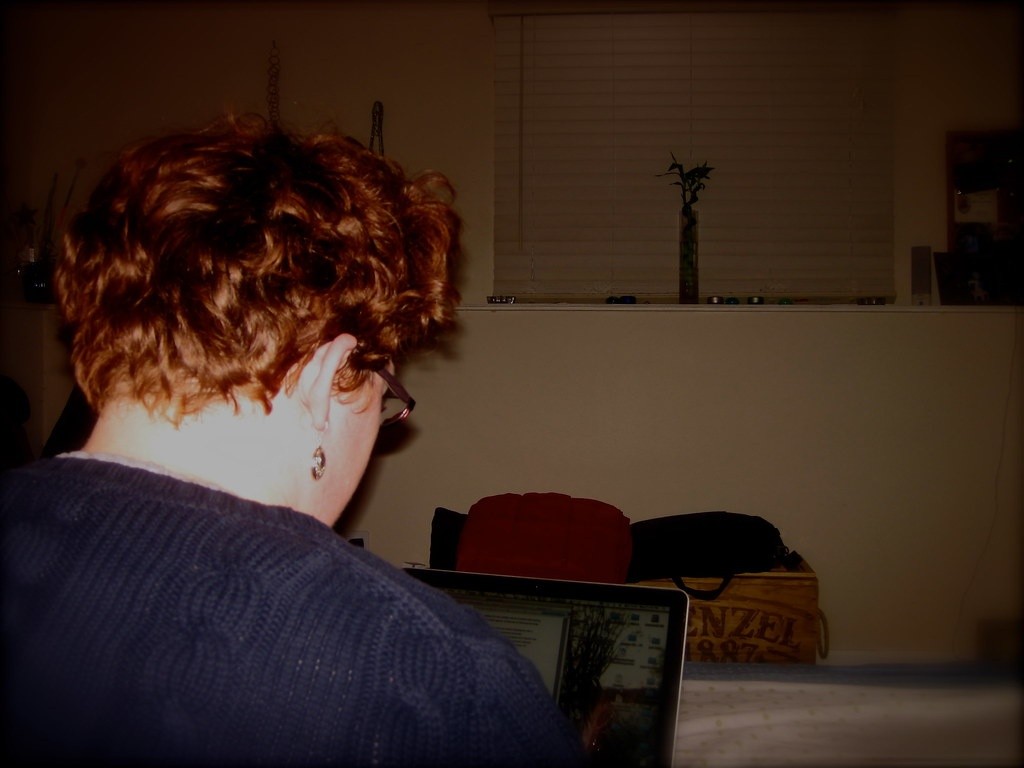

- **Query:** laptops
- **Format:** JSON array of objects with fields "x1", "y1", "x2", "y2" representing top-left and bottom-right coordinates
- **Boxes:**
[{"x1": 403, "y1": 566, "x2": 690, "y2": 768}]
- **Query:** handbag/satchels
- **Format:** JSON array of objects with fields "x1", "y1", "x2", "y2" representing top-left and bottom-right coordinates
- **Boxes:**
[
  {"x1": 625, "y1": 511, "x2": 788, "y2": 600},
  {"x1": 456, "y1": 492, "x2": 631, "y2": 584}
]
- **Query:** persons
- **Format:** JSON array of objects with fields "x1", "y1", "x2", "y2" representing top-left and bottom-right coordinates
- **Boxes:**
[{"x1": 0, "y1": 110, "x2": 573, "y2": 768}]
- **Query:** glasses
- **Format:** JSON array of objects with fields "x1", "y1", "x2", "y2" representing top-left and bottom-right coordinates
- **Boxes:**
[{"x1": 329, "y1": 335, "x2": 416, "y2": 428}]
